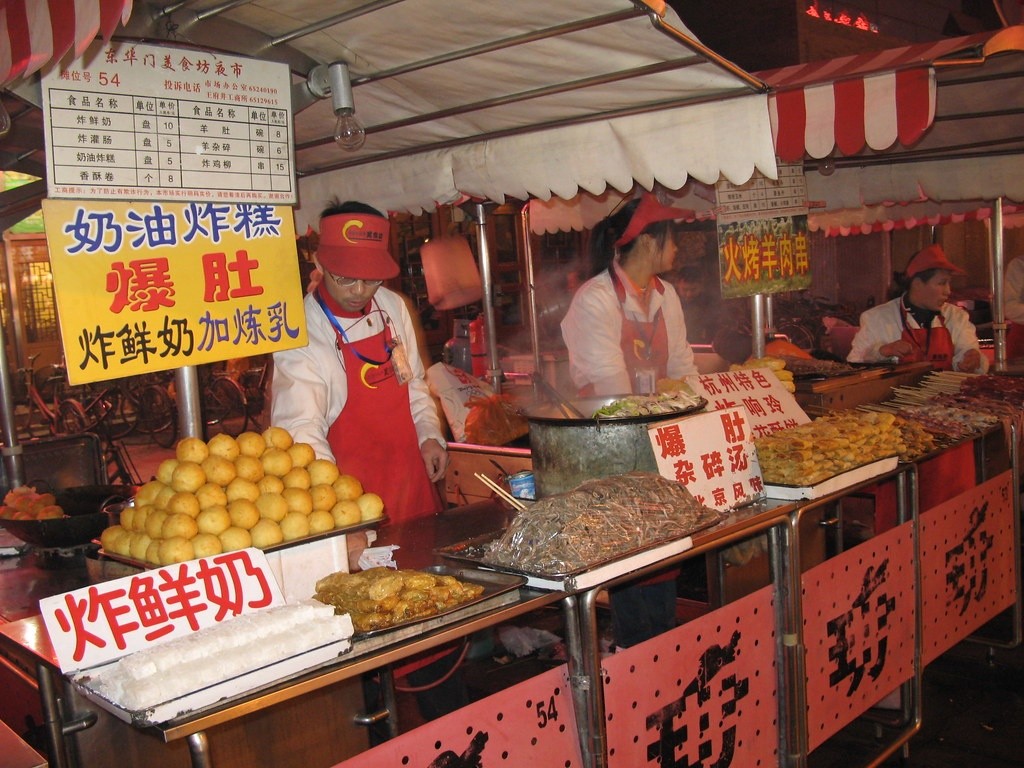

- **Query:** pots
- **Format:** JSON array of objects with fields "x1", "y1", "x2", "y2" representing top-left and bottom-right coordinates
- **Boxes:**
[
  {"x1": 516, "y1": 393, "x2": 708, "y2": 425},
  {"x1": 0, "y1": 479, "x2": 134, "y2": 548}
]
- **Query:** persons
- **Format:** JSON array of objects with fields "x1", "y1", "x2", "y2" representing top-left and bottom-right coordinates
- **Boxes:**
[
  {"x1": 561, "y1": 194, "x2": 702, "y2": 647},
  {"x1": 1004, "y1": 255, "x2": 1024, "y2": 359},
  {"x1": 676, "y1": 267, "x2": 718, "y2": 344},
  {"x1": 847, "y1": 246, "x2": 989, "y2": 533},
  {"x1": 957, "y1": 300, "x2": 974, "y2": 323},
  {"x1": 271, "y1": 200, "x2": 496, "y2": 721}
]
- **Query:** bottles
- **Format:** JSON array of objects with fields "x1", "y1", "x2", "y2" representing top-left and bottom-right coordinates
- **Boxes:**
[{"x1": 508, "y1": 470, "x2": 536, "y2": 506}]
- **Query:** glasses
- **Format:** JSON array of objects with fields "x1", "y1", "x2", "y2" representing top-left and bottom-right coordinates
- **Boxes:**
[{"x1": 328, "y1": 272, "x2": 384, "y2": 287}]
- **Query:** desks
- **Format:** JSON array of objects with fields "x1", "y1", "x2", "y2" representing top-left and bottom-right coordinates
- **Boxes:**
[{"x1": 510, "y1": 354, "x2": 569, "y2": 392}]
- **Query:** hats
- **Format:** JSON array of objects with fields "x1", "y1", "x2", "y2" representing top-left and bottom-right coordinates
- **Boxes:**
[
  {"x1": 315, "y1": 214, "x2": 400, "y2": 280},
  {"x1": 611, "y1": 192, "x2": 693, "y2": 250},
  {"x1": 905, "y1": 244, "x2": 965, "y2": 278}
]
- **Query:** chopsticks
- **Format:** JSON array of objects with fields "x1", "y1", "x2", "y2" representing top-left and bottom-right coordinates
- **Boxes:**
[{"x1": 473, "y1": 472, "x2": 525, "y2": 511}]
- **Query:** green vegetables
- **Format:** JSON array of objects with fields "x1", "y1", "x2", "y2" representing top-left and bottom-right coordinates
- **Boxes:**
[{"x1": 590, "y1": 400, "x2": 639, "y2": 418}]
